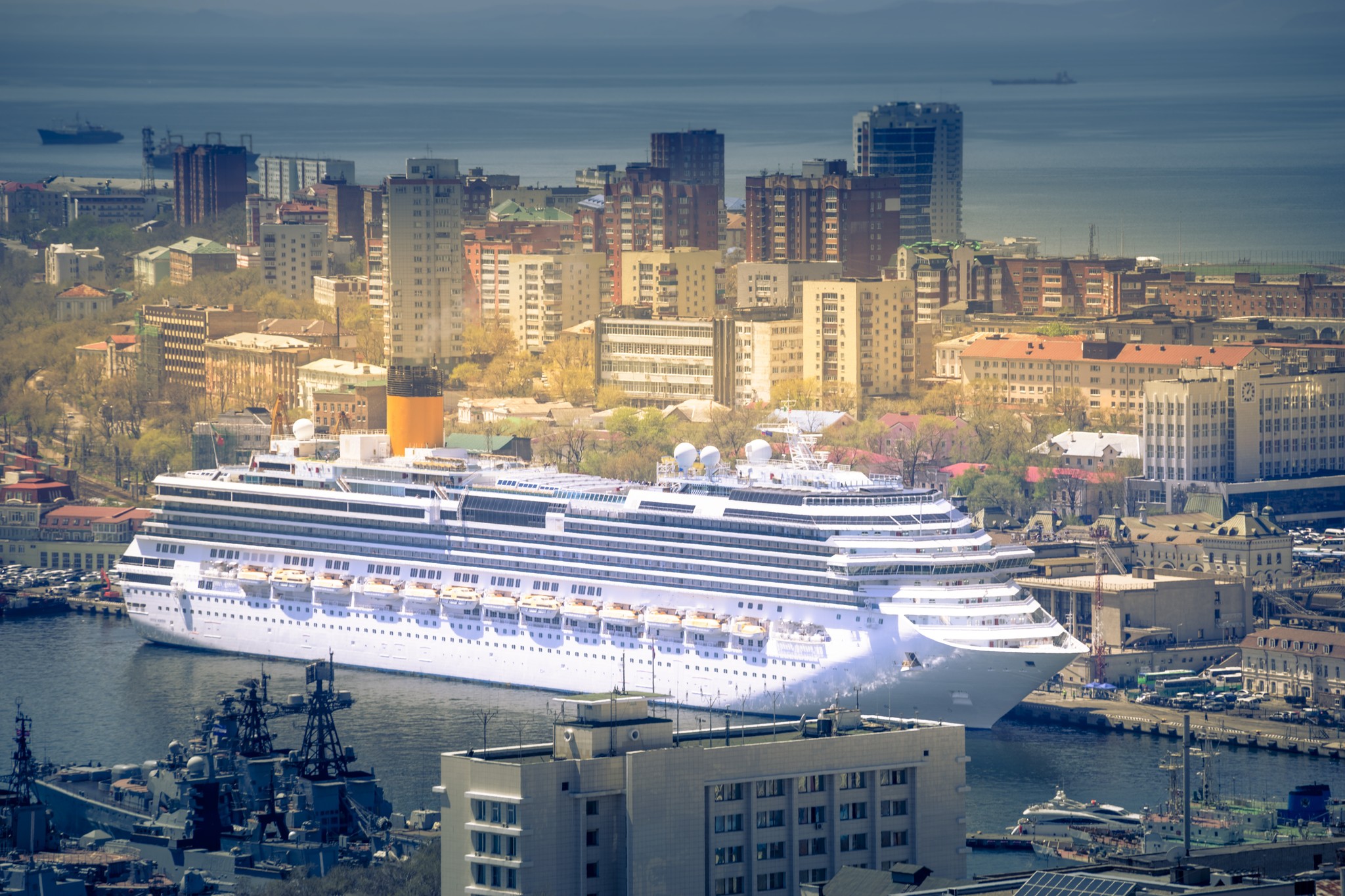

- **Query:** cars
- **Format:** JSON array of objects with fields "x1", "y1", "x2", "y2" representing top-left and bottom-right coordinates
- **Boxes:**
[
  {"x1": 67, "y1": 412, "x2": 75, "y2": 418},
  {"x1": 0, "y1": 563, "x2": 101, "y2": 599}
]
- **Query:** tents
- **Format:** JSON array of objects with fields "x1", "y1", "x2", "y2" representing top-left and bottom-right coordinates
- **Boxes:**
[
  {"x1": 1094, "y1": 682, "x2": 1118, "y2": 700},
  {"x1": 1081, "y1": 681, "x2": 1102, "y2": 699}
]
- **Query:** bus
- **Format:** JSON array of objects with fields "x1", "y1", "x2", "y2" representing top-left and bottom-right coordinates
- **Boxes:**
[
  {"x1": 1138, "y1": 666, "x2": 1244, "y2": 696},
  {"x1": 1292, "y1": 547, "x2": 1345, "y2": 568}
]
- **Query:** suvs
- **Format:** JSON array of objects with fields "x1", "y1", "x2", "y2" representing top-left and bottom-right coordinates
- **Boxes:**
[
  {"x1": 1286, "y1": 526, "x2": 1326, "y2": 546},
  {"x1": 1270, "y1": 710, "x2": 1299, "y2": 722},
  {"x1": 1167, "y1": 690, "x2": 1271, "y2": 712}
]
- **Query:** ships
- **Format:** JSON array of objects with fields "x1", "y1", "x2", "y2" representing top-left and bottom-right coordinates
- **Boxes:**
[{"x1": 111, "y1": 393, "x2": 1089, "y2": 732}]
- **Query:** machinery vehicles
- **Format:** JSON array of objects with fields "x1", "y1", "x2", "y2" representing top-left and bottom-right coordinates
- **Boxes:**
[{"x1": 99, "y1": 567, "x2": 121, "y2": 602}]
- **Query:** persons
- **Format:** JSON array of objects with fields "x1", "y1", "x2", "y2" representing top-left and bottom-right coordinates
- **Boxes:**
[
  {"x1": 1063, "y1": 683, "x2": 1148, "y2": 700},
  {"x1": 1083, "y1": 638, "x2": 1193, "y2": 654},
  {"x1": 1205, "y1": 713, "x2": 1208, "y2": 720}
]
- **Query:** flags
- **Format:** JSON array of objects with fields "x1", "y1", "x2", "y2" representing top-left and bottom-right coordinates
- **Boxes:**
[
  {"x1": 209, "y1": 422, "x2": 224, "y2": 446},
  {"x1": 1033, "y1": 507, "x2": 1036, "y2": 517},
  {"x1": 1053, "y1": 508, "x2": 1057, "y2": 516}
]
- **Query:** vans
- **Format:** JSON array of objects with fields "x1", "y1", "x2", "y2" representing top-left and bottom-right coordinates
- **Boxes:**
[
  {"x1": 1324, "y1": 528, "x2": 1345, "y2": 538},
  {"x1": 107, "y1": 569, "x2": 122, "y2": 581}
]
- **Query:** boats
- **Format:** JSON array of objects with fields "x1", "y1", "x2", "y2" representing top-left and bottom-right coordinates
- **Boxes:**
[
  {"x1": 1011, "y1": 776, "x2": 1149, "y2": 863},
  {"x1": 0, "y1": 645, "x2": 392, "y2": 896},
  {"x1": 234, "y1": 563, "x2": 768, "y2": 645}
]
